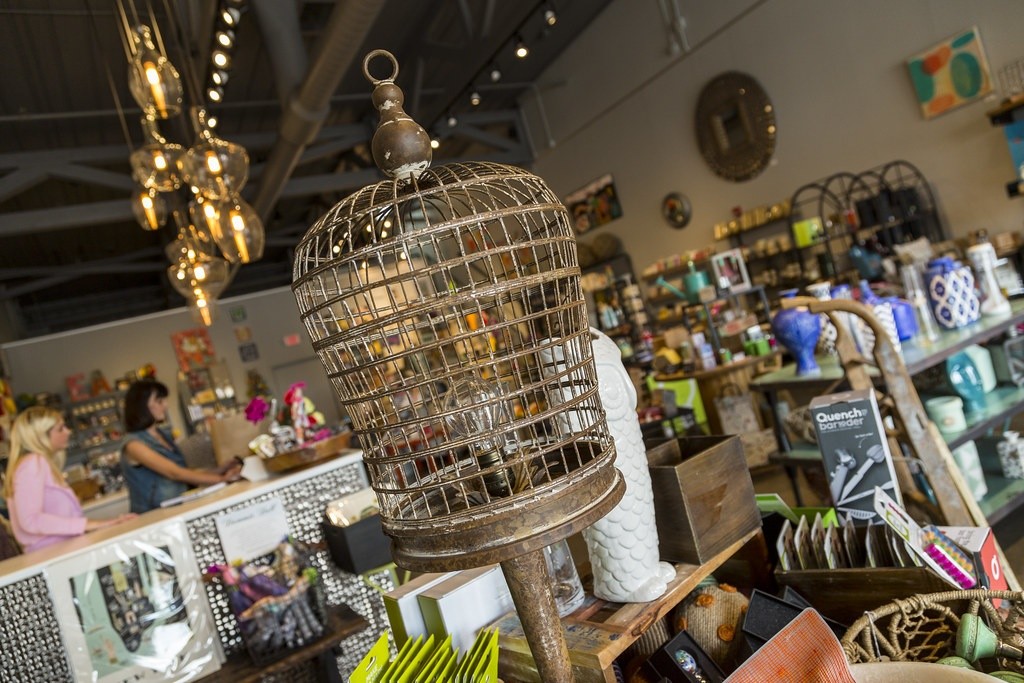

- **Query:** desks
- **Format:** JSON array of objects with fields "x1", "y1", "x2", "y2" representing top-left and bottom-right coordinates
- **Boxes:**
[
  {"x1": 654, "y1": 342, "x2": 794, "y2": 476},
  {"x1": 474, "y1": 526, "x2": 776, "y2": 683}
]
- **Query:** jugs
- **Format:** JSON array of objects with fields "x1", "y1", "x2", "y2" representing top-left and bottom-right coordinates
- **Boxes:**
[{"x1": 656, "y1": 261, "x2": 709, "y2": 305}]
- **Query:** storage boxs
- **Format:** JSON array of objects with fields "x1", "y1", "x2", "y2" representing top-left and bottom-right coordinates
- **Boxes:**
[
  {"x1": 641, "y1": 433, "x2": 763, "y2": 564},
  {"x1": 773, "y1": 520, "x2": 1010, "y2": 671},
  {"x1": 317, "y1": 510, "x2": 518, "y2": 664}
]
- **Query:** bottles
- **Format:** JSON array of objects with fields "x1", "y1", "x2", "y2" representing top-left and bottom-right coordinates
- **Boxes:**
[
  {"x1": 996, "y1": 430, "x2": 1024, "y2": 479},
  {"x1": 857, "y1": 279, "x2": 885, "y2": 308},
  {"x1": 773, "y1": 287, "x2": 822, "y2": 376},
  {"x1": 805, "y1": 281, "x2": 840, "y2": 357}
]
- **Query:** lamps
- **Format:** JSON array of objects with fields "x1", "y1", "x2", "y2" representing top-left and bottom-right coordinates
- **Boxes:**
[
  {"x1": 429, "y1": 0, "x2": 558, "y2": 150},
  {"x1": 91, "y1": 0, "x2": 265, "y2": 329}
]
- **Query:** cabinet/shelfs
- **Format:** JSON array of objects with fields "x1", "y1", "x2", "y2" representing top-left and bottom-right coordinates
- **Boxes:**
[{"x1": 747, "y1": 292, "x2": 1024, "y2": 552}]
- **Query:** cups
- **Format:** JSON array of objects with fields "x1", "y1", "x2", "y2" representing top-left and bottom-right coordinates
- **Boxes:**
[{"x1": 543, "y1": 539, "x2": 585, "y2": 618}]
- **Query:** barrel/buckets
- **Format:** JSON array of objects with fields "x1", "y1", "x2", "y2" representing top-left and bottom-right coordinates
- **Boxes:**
[{"x1": 925, "y1": 395, "x2": 967, "y2": 434}]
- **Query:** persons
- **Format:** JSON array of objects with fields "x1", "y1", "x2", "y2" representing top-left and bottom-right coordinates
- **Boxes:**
[
  {"x1": 2, "y1": 407, "x2": 136, "y2": 553},
  {"x1": 120, "y1": 380, "x2": 244, "y2": 514}
]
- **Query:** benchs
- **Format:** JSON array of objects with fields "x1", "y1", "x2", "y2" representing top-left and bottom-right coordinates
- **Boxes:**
[{"x1": 190, "y1": 602, "x2": 372, "y2": 683}]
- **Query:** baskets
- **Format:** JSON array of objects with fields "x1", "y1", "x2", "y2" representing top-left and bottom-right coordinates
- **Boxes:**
[{"x1": 839, "y1": 590, "x2": 1024, "y2": 673}]
